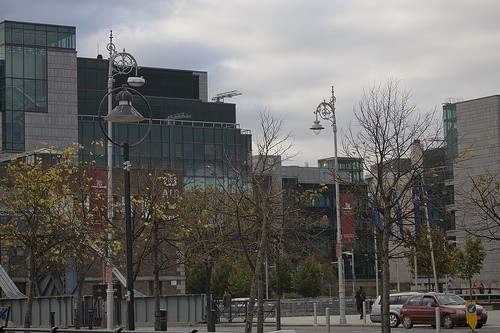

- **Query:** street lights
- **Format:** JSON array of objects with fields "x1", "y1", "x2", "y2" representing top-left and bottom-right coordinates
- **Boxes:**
[
  {"x1": 103, "y1": 28, "x2": 146, "y2": 333},
  {"x1": 308, "y1": 85, "x2": 347, "y2": 326},
  {"x1": 97, "y1": 83, "x2": 154, "y2": 330},
  {"x1": 342, "y1": 248, "x2": 355, "y2": 315}
]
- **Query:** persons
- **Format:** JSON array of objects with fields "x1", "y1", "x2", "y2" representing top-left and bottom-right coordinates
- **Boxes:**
[
  {"x1": 355, "y1": 286, "x2": 366, "y2": 319},
  {"x1": 223, "y1": 289, "x2": 232, "y2": 322}
]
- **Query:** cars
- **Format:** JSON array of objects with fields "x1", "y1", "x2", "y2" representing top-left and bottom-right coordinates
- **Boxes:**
[
  {"x1": 369, "y1": 291, "x2": 421, "y2": 328},
  {"x1": 398, "y1": 291, "x2": 488, "y2": 329}
]
